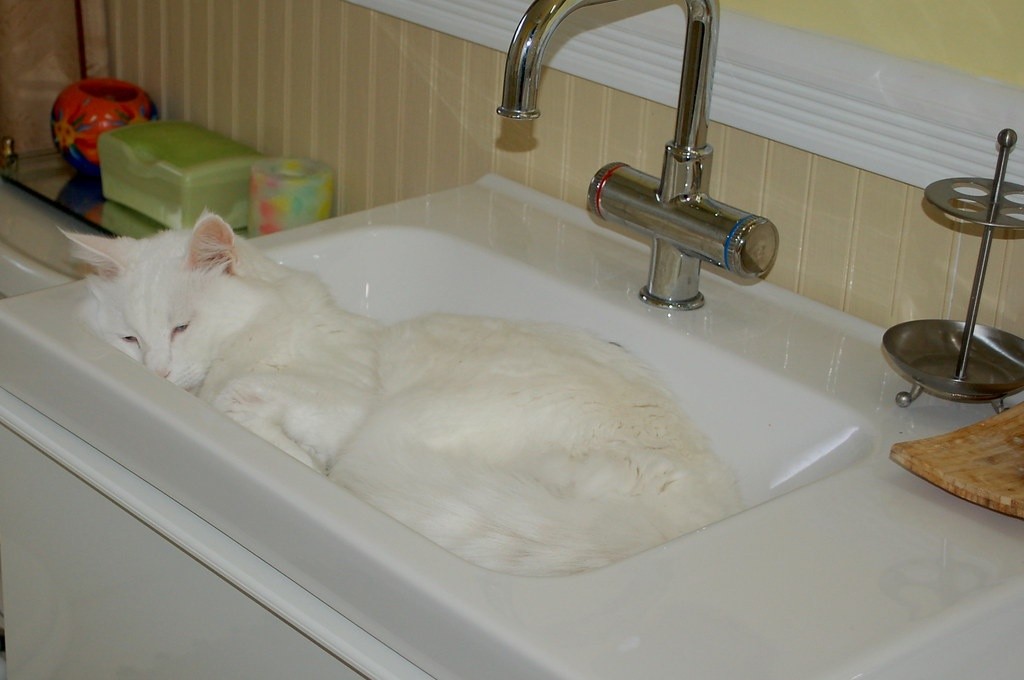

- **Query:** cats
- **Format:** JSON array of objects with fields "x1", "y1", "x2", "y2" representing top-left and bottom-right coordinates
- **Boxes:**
[{"x1": 58, "y1": 211, "x2": 746, "y2": 576}]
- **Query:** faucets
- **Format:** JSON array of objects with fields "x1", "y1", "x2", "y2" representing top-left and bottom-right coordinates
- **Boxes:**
[{"x1": 496, "y1": 0, "x2": 781, "y2": 310}]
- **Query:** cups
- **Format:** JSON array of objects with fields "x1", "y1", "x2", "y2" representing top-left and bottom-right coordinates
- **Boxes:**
[{"x1": 246, "y1": 156, "x2": 336, "y2": 238}]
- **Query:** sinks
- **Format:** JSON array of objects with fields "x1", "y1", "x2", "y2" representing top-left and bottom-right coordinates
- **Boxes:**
[{"x1": 0, "y1": 226, "x2": 877, "y2": 622}]
